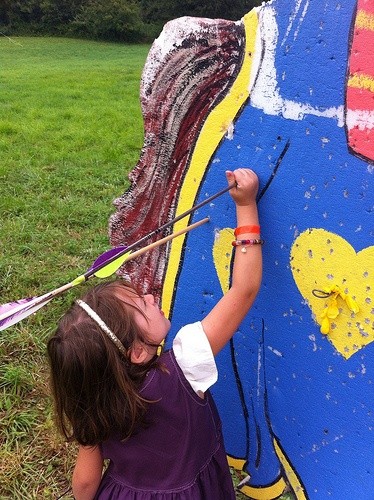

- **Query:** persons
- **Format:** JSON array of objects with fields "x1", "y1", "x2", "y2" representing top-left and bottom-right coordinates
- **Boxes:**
[{"x1": 46, "y1": 167, "x2": 263, "y2": 500}]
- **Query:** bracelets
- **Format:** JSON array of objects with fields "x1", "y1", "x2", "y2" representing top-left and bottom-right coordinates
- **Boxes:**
[
  {"x1": 234, "y1": 225, "x2": 261, "y2": 236},
  {"x1": 231, "y1": 240, "x2": 264, "y2": 252}
]
